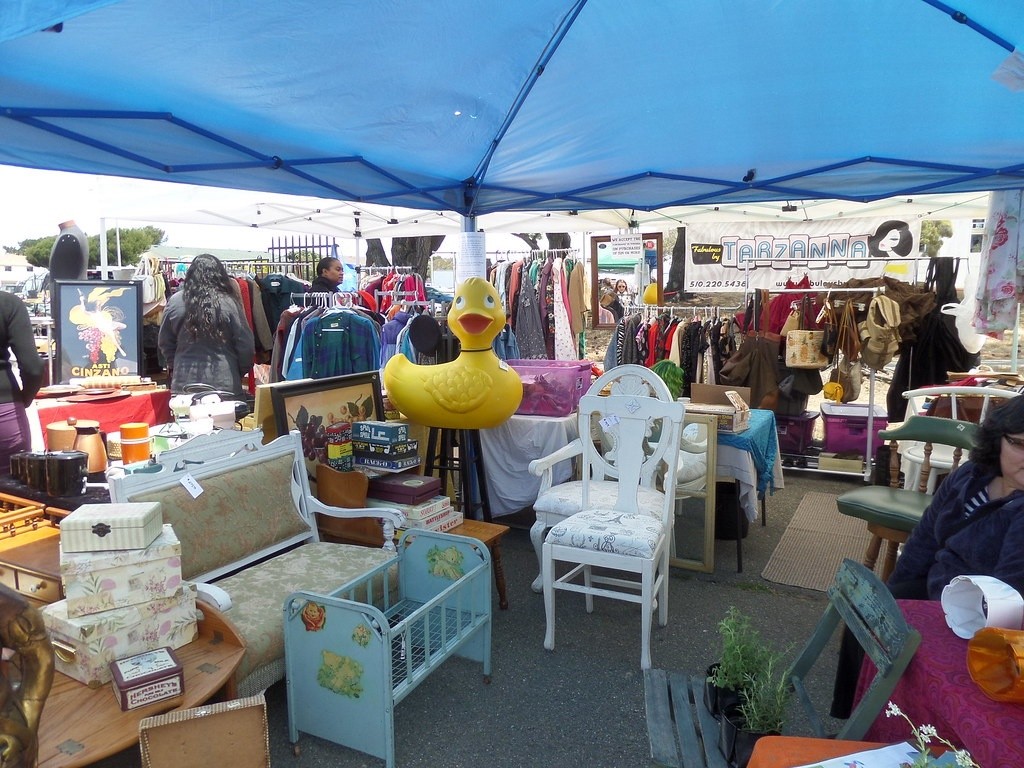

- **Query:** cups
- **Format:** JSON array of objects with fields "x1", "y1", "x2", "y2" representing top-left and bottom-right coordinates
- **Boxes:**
[
  {"x1": 120, "y1": 422, "x2": 151, "y2": 465},
  {"x1": 9, "y1": 447, "x2": 89, "y2": 498}
]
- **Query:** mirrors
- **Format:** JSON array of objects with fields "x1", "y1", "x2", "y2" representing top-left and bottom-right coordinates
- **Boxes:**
[
  {"x1": 600, "y1": 413, "x2": 718, "y2": 573},
  {"x1": 591, "y1": 232, "x2": 663, "y2": 330}
]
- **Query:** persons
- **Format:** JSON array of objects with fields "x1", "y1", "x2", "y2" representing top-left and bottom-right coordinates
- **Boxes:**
[
  {"x1": 48, "y1": 219, "x2": 90, "y2": 341},
  {"x1": 157, "y1": 252, "x2": 255, "y2": 419},
  {"x1": 0, "y1": 290, "x2": 45, "y2": 479},
  {"x1": 599, "y1": 276, "x2": 631, "y2": 322},
  {"x1": 830, "y1": 394, "x2": 1024, "y2": 716},
  {"x1": 306, "y1": 256, "x2": 345, "y2": 308}
]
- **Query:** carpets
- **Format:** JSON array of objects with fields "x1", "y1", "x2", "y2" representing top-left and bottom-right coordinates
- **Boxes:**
[{"x1": 761, "y1": 491, "x2": 897, "y2": 592}]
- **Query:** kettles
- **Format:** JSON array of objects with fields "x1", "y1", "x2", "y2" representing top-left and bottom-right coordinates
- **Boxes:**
[{"x1": 73, "y1": 427, "x2": 109, "y2": 483}]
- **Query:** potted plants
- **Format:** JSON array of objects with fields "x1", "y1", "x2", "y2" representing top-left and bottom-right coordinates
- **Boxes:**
[{"x1": 703, "y1": 607, "x2": 799, "y2": 768}]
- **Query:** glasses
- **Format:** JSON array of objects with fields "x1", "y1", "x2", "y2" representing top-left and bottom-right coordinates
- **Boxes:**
[{"x1": 1002, "y1": 431, "x2": 1024, "y2": 453}]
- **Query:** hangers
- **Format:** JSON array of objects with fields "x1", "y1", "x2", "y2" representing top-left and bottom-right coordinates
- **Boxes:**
[
  {"x1": 367, "y1": 266, "x2": 412, "y2": 278},
  {"x1": 495, "y1": 247, "x2": 581, "y2": 266},
  {"x1": 790, "y1": 290, "x2": 852, "y2": 323},
  {"x1": 290, "y1": 291, "x2": 429, "y2": 317},
  {"x1": 222, "y1": 262, "x2": 291, "y2": 279},
  {"x1": 624, "y1": 304, "x2": 737, "y2": 332}
]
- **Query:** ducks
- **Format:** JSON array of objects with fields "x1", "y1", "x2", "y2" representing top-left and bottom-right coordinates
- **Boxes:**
[{"x1": 381, "y1": 276, "x2": 524, "y2": 431}]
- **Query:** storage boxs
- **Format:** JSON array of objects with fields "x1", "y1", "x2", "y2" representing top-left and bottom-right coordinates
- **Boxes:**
[
  {"x1": 775, "y1": 411, "x2": 820, "y2": 454},
  {"x1": 325, "y1": 420, "x2": 463, "y2": 542},
  {"x1": 683, "y1": 383, "x2": 752, "y2": 434},
  {"x1": 37, "y1": 502, "x2": 200, "y2": 711},
  {"x1": 821, "y1": 403, "x2": 889, "y2": 458}
]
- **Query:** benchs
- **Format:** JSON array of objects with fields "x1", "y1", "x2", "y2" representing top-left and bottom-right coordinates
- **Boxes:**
[{"x1": 108, "y1": 428, "x2": 406, "y2": 702}]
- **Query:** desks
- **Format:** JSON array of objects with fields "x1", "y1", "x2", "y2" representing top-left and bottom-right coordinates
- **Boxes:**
[
  {"x1": 715, "y1": 409, "x2": 785, "y2": 573},
  {"x1": 850, "y1": 599, "x2": 1024, "y2": 768},
  {"x1": 35, "y1": 389, "x2": 175, "y2": 452},
  {"x1": 392, "y1": 520, "x2": 510, "y2": 610}
]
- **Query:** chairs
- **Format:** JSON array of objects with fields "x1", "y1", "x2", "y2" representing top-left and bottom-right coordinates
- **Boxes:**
[
  {"x1": 528, "y1": 364, "x2": 676, "y2": 612},
  {"x1": 897, "y1": 386, "x2": 1022, "y2": 557},
  {"x1": 836, "y1": 414, "x2": 979, "y2": 584},
  {"x1": 542, "y1": 395, "x2": 685, "y2": 670},
  {"x1": 644, "y1": 558, "x2": 922, "y2": 768}
]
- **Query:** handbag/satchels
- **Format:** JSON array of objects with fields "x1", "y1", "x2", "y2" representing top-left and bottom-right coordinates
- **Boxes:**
[
  {"x1": 720, "y1": 288, "x2": 901, "y2": 416},
  {"x1": 941, "y1": 259, "x2": 986, "y2": 354}
]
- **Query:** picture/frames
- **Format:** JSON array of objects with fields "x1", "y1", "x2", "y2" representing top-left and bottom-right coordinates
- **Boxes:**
[
  {"x1": 53, "y1": 278, "x2": 144, "y2": 387},
  {"x1": 270, "y1": 370, "x2": 385, "y2": 498}
]
